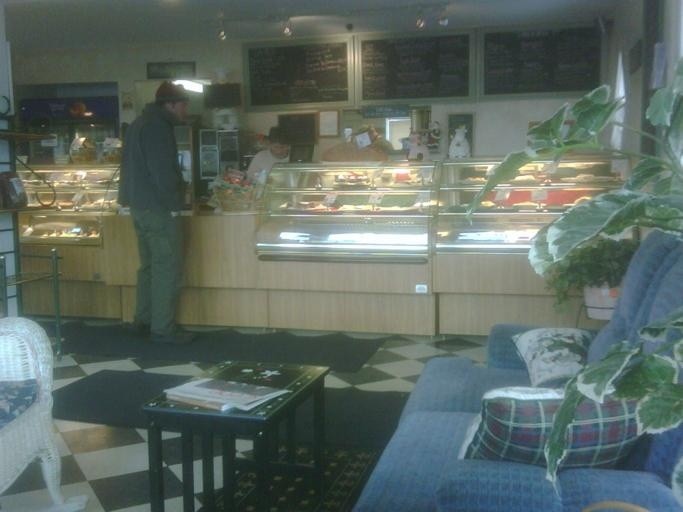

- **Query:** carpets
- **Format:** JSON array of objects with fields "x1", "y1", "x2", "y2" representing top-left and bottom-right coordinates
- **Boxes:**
[{"x1": 193, "y1": 440, "x2": 383, "y2": 510}]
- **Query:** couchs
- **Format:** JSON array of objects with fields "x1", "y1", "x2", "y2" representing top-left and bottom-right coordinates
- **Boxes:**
[{"x1": 348, "y1": 225, "x2": 683, "y2": 511}]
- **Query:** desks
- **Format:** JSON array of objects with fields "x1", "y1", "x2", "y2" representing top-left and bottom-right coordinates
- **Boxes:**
[{"x1": 136, "y1": 357, "x2": 330, "y2": 512}]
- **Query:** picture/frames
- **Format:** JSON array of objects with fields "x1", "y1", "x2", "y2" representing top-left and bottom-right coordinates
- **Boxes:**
[
  {"x1": 318, "y1": 109, "x2": 340, "y2": 139},
  {"x1": 446, "y1": 112, "x2": 475, "y2": 158}
]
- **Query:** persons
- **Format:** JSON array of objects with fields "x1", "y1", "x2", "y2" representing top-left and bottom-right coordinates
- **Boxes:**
[
  {"x1": 120, "y1": 82, "x2": 199, "y2": 348},
  {"x1": 246, "y1": 126, "x2": 293, "y2": 182}
]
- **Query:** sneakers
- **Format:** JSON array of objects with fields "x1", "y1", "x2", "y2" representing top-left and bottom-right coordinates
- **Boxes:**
[{"x1": 130, "y1": 320, "x2": 193, "y2": 345}]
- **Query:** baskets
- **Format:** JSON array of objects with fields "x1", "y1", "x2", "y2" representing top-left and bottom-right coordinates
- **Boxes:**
[
  {"x1": 69, "y1": 148, "x2": 97, "y2": 164},
  {"x1": 214, "y1": 188, "x2": 255, "y2": 212}
]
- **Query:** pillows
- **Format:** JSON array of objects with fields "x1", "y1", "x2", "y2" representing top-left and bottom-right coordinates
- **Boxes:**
[
  {"x1": 507, "y1": 328, "x2": 595, "y2": 389},
  {"x1": 458, "y1": 383, "x2": 652, "y2": 470}
]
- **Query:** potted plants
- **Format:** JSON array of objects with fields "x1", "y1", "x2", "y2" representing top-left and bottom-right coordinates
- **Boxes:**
[{"x1": 546, "y1": 235, "x2": 640, "y2": 322}]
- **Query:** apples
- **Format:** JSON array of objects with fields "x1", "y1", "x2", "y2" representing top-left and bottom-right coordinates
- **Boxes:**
[{"x1": 222, "y1": 171, "x2": 256, "y2": 198}]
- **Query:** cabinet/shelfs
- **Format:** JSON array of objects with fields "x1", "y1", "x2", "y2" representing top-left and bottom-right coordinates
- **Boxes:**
[
  {"x1": 254, "y1": 162, "x2": 436, "y2": 334},
  {"x1": 1, "y1": 95, "x2": 66, "y2": 362},
  {"x1": 15, "y1": 164, "x2": 121, "y2": 319},
  {"x1": 99, "y1": 211, "x2": 269, "y2": 331},
  {"x1": 432, "y1": 158, "x2": 626, "y2": 334}
]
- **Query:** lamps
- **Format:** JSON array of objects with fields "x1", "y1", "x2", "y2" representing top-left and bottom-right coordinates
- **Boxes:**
[{"x1": 211, "y1": 1, "x2": 451, "y2": 42}]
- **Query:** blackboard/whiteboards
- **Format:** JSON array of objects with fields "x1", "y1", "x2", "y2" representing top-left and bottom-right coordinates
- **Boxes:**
[
  {"x1": 353, "y1": 33, "x2": 477, "y2": 106},
  {"x1": 476, "y1": 27, "x2": 608, "y2": 100},
  {"x1": 242, "y1": 35, "x2": 354, "y2": 112}
]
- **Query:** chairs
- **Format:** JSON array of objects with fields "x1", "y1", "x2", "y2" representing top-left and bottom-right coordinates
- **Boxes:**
[{"x1": 0, "y1": 315, "x2": 71, "y2": 512}]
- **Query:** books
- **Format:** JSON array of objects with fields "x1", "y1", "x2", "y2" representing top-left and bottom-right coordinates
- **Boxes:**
[
  {"x1": 163, "y1": 376, "x2": 289, "y2": 411},
  {"x1": 165, "y1": 392, "x2": 234, "y2": 412}
]
